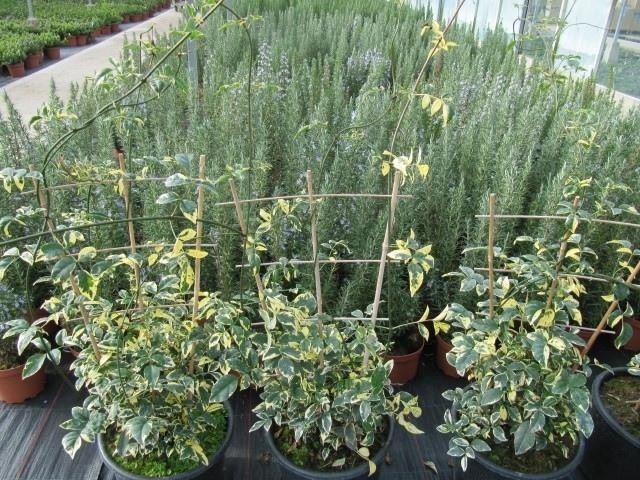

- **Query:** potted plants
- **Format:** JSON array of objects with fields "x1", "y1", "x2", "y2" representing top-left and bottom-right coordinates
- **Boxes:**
[{"x1": 0, "y1": 0, "x2": 640, "y2": 480}]
